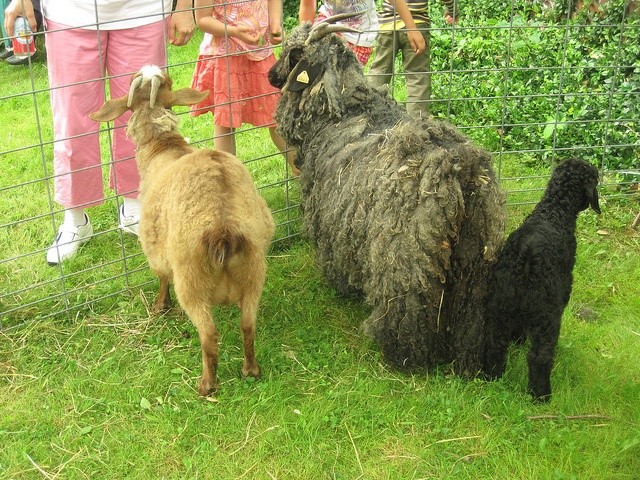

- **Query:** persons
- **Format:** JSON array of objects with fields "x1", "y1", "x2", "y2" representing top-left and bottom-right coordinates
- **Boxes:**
[
  {"x1": 190, "y1": 0, "x2": 304, "y2": 176},
  {"x1": 365, "y1": 0, "x2": 459, "y2": 115},
  {"x1": 298, "y1": 0, "x2": 426, "y2": 69},
  {"x1": 4, "y1": 0, "x2": 196, "y2": 266}
]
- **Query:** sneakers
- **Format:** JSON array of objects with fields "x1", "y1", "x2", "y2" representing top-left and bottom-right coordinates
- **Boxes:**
[
  {"x1": 47, "y1": 212, "x2": 94, "y2": 265},
  {"x1": 119, "y1": 205, "x2": 140, "y2": 237}
]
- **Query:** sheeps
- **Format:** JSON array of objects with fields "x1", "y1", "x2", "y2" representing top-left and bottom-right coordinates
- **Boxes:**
[
  {"x1": 400, "y1": 196, "x2": 412, "y2": 205},
  {"x1": 269, "y1": 9, "x2": 509, "y2": 383},
  {"x1": 478, "y1": 158, "x2": 601, "y2": 405},
  {"x1": 89, "y1": 65, "x2": 276, "y2": 396}
]
